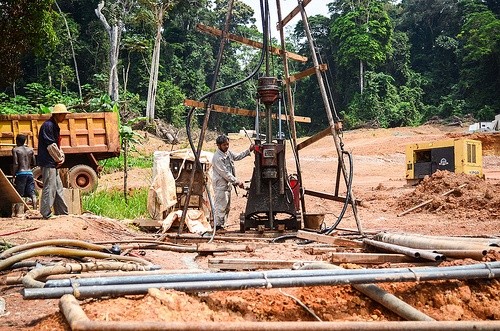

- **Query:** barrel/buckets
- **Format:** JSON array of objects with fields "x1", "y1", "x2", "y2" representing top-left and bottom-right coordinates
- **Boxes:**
[{"x1": 303, "y1": 213, "x2": 325, "y2": 228}]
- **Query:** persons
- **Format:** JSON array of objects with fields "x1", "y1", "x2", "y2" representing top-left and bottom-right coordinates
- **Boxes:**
[
  {"x1": 11, "y1": 134, "x2": 36, "y2": 217},
  {"x1": 211, "y1": 135, "x2": 254, "y2": 231},
  {"x1": 36, "y1": 104, "x2": 71, "y2": 220}
]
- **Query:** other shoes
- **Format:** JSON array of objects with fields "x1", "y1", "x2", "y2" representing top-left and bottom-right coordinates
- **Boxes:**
[{"x1": 217, "y1": 225, "x2": 227, "y2": 232}]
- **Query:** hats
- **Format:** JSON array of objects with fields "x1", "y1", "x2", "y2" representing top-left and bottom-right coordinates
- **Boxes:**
[{"x1": 51, "y1": 104, "x2": 71, "y2": 114}]
